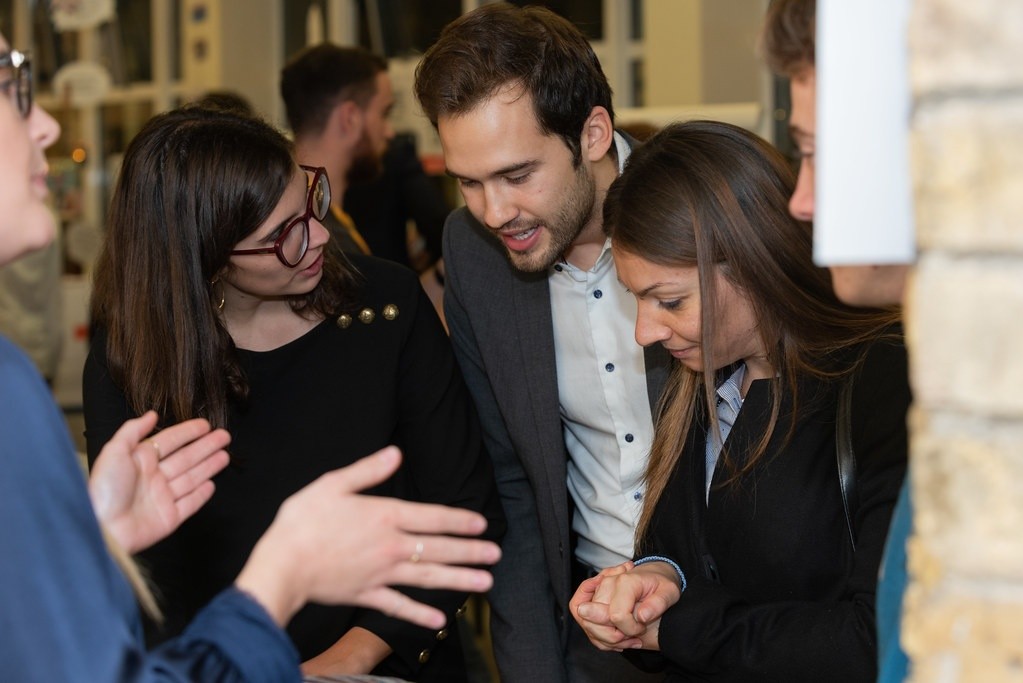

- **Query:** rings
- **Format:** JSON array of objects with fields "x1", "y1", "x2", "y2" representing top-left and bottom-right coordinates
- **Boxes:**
[{"x1": 407, "y1": 534, "x2": 423, "y2": 563}]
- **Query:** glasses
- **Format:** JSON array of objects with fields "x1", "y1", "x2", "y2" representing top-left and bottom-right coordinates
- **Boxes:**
[
  {"x1": 225, "y1": 164, "x2": 332, "y2": 268},
  {"x1": 0, "y1": 51, "x2": 33, "y2": 119}
]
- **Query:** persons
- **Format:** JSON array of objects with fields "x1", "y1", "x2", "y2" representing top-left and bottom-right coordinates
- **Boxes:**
[
  {"x1": 0, "y1": 32, "x2": 502, "y2": 681},
  {"x1": 276, "y1": 41, "x2": 398, "y2": 278},
  {"x1": 752, "y1": 2, "x2": 916, "y2": 683},
  {"x1": 567, "y1": 125, "x2": 913, "y2": 683},
  {"x1": 415, "y1": 4, "x2": 738, "y2": 683},
  {"x1": 80, "y1": 102, "x2": 502, "y2": 683}
]
